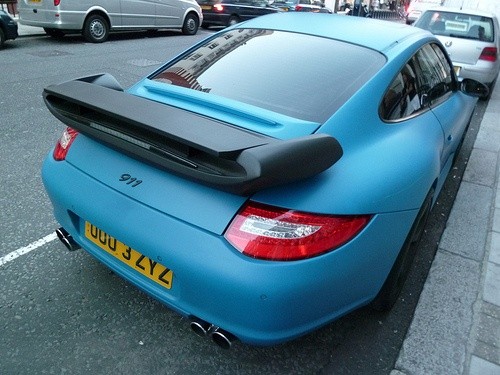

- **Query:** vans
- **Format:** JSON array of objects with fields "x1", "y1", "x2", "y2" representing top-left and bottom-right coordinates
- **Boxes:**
[{"x1": 17, "y1": 0, "x2": 204, "y2": 43}]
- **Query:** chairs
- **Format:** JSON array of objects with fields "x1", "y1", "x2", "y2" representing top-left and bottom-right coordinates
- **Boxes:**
[
  {"x1": 469, "y1": 24, "x2": 485, "y2": 38},
  {"x1": 432, "y1": 21, "x2": 446, "y2": 31}
]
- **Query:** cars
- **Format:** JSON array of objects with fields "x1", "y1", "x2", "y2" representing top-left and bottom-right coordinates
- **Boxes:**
[
  {"x1": 411, "y1": 8, "x2": 500, "y2": 85},
  {"x1": 196, "y1": 0, "x2": 333, "y2": 29}
]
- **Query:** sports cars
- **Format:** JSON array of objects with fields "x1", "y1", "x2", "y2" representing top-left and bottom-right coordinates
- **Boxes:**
[{"x1": 42, "y1": 12, "x2": 489, "y2": 352}]
